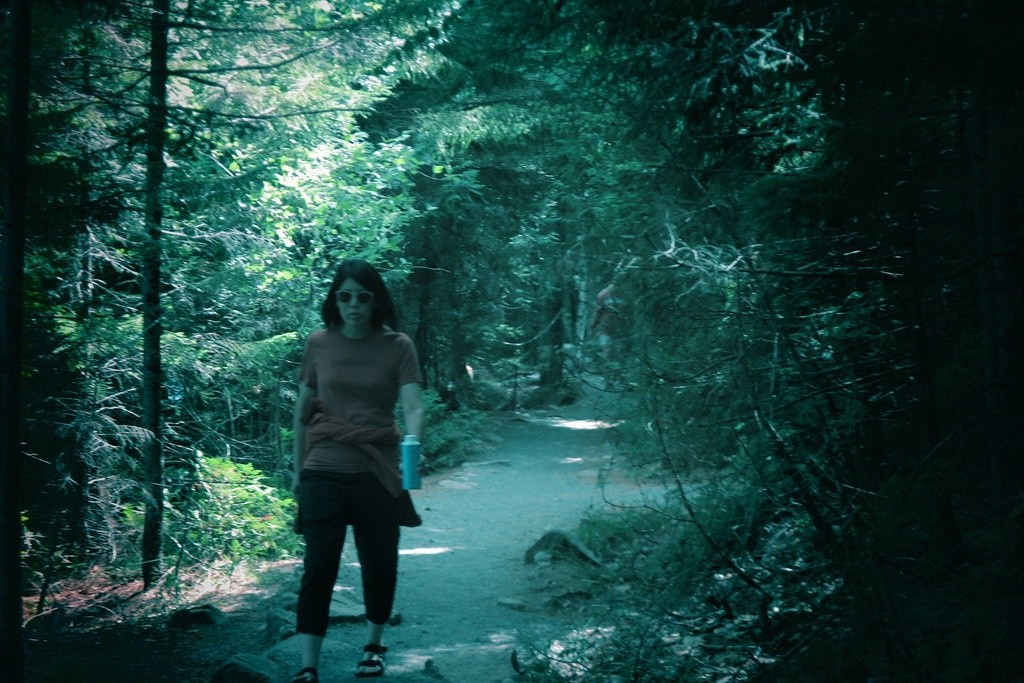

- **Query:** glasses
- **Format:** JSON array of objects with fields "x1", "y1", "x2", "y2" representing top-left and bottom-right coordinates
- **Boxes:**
[{"x1": 336, "y1": 290, "x2": 374, "y2": 305}]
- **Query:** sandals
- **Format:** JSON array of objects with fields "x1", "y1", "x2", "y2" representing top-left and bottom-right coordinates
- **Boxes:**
[
  {"x1": 291, "y1": 667, "x2": 317, "y2": 683},
  {"x1": 356, "y1": 644, "x2": 388, "y2": 677}
]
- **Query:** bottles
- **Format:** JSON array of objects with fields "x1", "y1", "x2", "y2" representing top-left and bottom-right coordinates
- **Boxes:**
[{"x1": 401, "y1": 434, "x2": 421, "y2": 490}]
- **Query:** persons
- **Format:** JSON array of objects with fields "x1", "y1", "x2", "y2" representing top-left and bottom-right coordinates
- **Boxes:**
[
  {"x1": 291, "y1": 258, "x2": 426, "y2": 683},
  {"x1": 592, "y1": 281, "x2": 636, "y2": 392}
]
